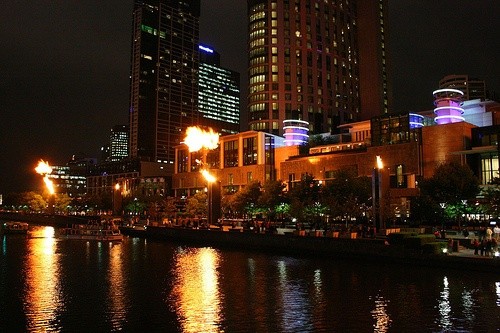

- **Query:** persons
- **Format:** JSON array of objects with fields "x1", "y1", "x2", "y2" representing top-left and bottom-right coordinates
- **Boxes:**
[
  {"x1": 480, "y1": 238, "x2": 497, "y2": 257},
  {"x1": 474, "y1": 239, "x2": 480, "y2": 255}
]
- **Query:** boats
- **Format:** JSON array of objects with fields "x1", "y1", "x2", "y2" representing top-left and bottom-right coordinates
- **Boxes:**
[
  {"x1": 62, "y1": 222, "x2": 123, "y2": 242},
  {"x1": 0, "y1": 220, "x2": 29, "y2": 234},
  {"x1": 119, "y1": 225, "x2": 157, "y2": 238}
]
